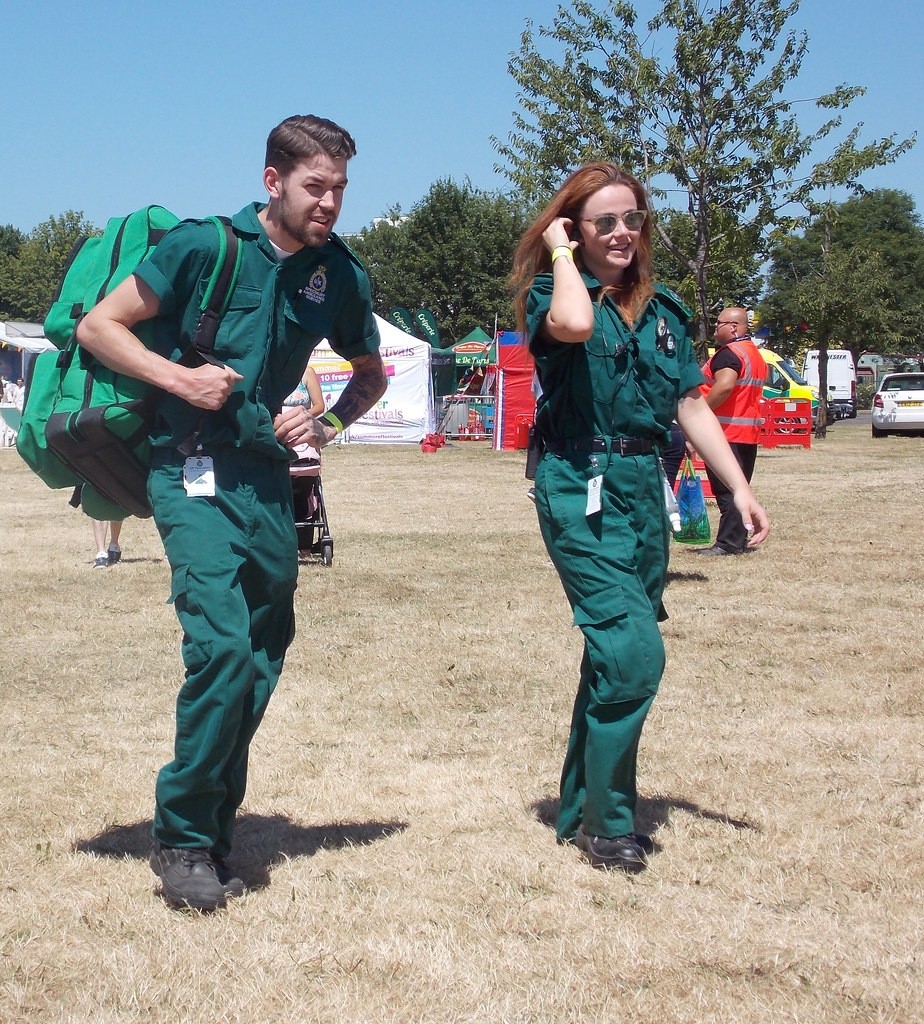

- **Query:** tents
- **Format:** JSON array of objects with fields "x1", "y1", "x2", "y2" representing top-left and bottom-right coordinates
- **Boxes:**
[{"x1": 0, "y1": 314, "x2": 540, "y2": 452}]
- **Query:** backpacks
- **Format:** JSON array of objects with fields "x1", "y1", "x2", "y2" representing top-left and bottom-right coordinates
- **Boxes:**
[{"x1": 15, "y1": 205, "x2": 243, "y2": 521}]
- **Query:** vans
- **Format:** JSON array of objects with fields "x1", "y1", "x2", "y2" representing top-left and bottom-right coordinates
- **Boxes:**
[
  {"x1": 801, "y1": 349, "x2": 856, "y2": 418},
  {"x1": 706, "y1": 346, "x2": 838, "y2": 433}
]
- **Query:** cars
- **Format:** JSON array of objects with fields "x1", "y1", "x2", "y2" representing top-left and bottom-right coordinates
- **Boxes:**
[{"x1": 870, "y1": 372, "x2": 924, "y2": 438}]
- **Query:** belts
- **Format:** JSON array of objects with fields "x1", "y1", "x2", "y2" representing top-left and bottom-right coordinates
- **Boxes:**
[{"x1": 556, "y1": 436, "x2": 656, "y2": 458}]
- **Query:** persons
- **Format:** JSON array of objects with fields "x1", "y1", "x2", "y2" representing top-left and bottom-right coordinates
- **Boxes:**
[
  {"x1": 0, "y1": 374, "x2": 122, "y2": 570},
  {"x1": 282, "y1": 365, "x2": 325, "y2": 414},
  {"x1": 692, "y1": 308, "x2": 769, "y2": 555},
  {"x1": 507, "y1": 165, "x2": 771, "y2": 872},
  {"x1": 78, "y1": 114, "x2": 388, "y2": 912}
]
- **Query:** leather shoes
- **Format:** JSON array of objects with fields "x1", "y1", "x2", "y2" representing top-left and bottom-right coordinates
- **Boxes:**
[
  {"x1": 149, "y1": 841, "x2": 247, "y2": 909},
  {"x1": 576, "y1": 823, "x2": 654, "y2": 868}
]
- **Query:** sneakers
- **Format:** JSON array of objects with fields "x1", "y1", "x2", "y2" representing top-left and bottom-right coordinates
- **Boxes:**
[
  {"x1": 108, "y1": 550, "x2": 122, "y2": 565},
  {"x1": 93, "y1": 556, "x2": 109, "y2": 570}
]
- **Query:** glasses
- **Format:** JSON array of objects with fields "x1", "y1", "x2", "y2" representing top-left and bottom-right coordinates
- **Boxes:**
[
  {"x1": 717, "y1": 320, "x2": 738, "y2": 324},
  {"x1": 579, "y1": 210, "x2": 647, "y2": 235}
]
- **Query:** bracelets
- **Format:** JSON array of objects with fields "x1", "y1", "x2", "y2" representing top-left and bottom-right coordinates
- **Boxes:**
[
  {"x1": 323, "y1": 409, "x2": 345, "y2": 432},
  {"x1": 550, "y1": 244, "x2": 573, "y2": 262}
]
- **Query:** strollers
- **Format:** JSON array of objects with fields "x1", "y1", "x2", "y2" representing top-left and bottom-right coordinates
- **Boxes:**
[{"x1": 289, "y1": 452, "x2": 334, "y2": 567}]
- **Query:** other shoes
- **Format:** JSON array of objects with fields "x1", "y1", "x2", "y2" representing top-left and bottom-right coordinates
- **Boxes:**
[{"x1": 697, "y1": 545, "x2": 743, "y2": 556}]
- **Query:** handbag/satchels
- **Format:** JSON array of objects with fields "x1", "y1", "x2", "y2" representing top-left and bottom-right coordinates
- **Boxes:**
[{"x1": 674, "y1": 457, "x2": 711, "y2": 544}]
